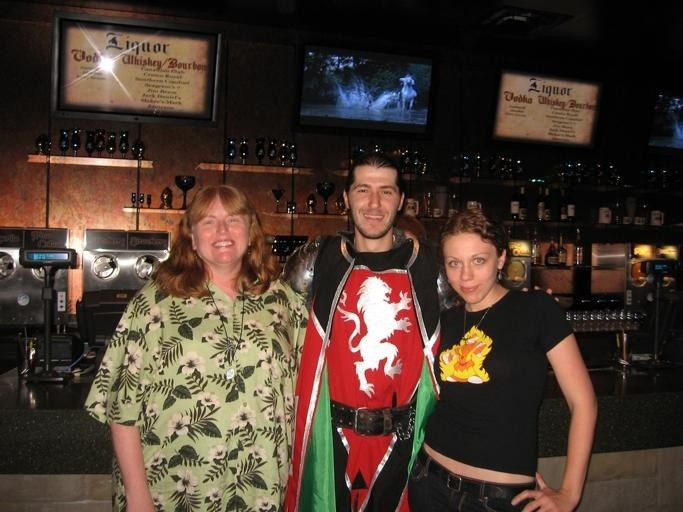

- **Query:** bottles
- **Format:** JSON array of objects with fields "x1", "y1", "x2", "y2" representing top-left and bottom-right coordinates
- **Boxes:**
[
  {"x1": 404, "y1": 179, "x2": 682, "y2": 230},
  {"x1": 526, "y1": 222, "x2": 584, "y2": 268},
  {"x1": 268, "y1": 239, "x2": 306, "y2": 265}
]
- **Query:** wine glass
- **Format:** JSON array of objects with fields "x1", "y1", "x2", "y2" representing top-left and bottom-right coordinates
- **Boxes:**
[
  {"x1": 270, "y1": 179, "x2": 347, "y2": 216},
  {"x1": 227, "y1": 135, "x2": 299, "y2": 168},
  {"x1": 34, "y1": 125, "x2": 147, "y2": 162},
  {"x1": 349, "y1": 139, "x2": 428, "y2": 177},
  {"x1": 174, "y1": 174, "x2": 197, "y2": 209},
  {"x1": 131, "y1": 192, "x2": 153, "y2": 208},
  {"x1": 449, "y1": 150, "x2": 526, "y2": 182},
  {"x1": 545, "y1": 159, "x2": 683, "y2": 190}
]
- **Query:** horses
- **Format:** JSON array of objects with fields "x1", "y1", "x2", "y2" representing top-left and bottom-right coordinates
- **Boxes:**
[{"x1": 398, "y1": 76, "x2": 417, "y2": 112}]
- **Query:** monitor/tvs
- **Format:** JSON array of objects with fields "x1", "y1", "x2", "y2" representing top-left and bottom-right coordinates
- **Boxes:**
[
  {"x1": 49, "y1": 10, "x2": 222, "y2": 129},
  {"x1": 644, "y1": 86, "x2": 683, "y2": 158},
  {"x1": 488, "y1": 69, "x2": 601, "y2": 147},
  {"x1": 76, "y1": 289, "x2": 140, "y2": 346},
  {"x1": 297, "y1": 43, "x2": 433, "y2": 135}
]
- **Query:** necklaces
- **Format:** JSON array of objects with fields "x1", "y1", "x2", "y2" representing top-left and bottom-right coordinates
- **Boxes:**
[
  {"x1": 205, "y1": 280, "x2": 245, "y2": 379},
  {"x1": 462, "y1": 291, "x2": 501, "y2": 340}
]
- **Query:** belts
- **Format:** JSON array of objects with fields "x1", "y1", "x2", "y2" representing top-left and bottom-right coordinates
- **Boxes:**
[
  {"x1": 418, "y1": 447, "x2": 537, "y2": 501},
  {"x1": 328, "y1": 400, "x2": 411, "y2": 437}
]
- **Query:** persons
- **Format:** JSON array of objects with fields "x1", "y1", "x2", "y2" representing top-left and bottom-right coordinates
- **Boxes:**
[
  {"x1": 408, "y1": 208, "x2": 599, "y2": 512},
  {"x1": 84, "y1": 183, "x2": 309, "y2": 512},
  {"x1": 279, "y1": 150, "x2": 458, "y2": 511}
]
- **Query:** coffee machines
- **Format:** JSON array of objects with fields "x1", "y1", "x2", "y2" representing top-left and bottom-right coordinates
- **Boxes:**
[
  {"x1": 0, "y1": 224, "x2": 70, "y2": 373},
  {"x1": 623, "y1": 255, "x2": 680, "y2": 374},
  {"x1": 78, "y1": 226, "x2": 172, "y2": 358}
]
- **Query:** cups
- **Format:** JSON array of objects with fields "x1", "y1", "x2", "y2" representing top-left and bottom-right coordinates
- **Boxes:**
[
  {"x1": 562, "y1": 308, "x2": 642, "y2": 322},
  {"x1": 569, "y1": 322, "x2": 641, "y2": 332}
]
